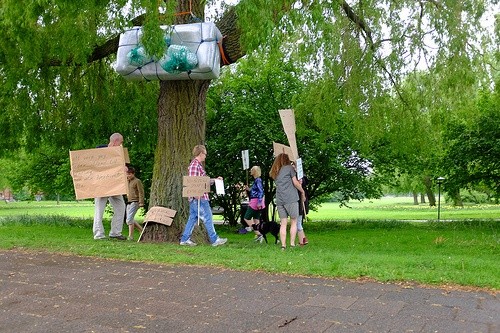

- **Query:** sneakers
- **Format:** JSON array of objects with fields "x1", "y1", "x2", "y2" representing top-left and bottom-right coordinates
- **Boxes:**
[
  {"x1": 212, "y1": 236, "x2": 227, "y2": 247},
  {"x1": 254, "y1": 234, "x2": 263, "y2": 243},
  {"x1": 110, "y1": 235, "x2": 126, "y2": 240},
  {"x1": 180, "y1": 238, "x2": 197, "y2": 246}
]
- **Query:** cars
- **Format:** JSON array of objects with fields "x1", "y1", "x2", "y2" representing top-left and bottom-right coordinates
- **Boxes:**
[{"x1": 211, "y1": 185, "x2": 236, "y2": 215}]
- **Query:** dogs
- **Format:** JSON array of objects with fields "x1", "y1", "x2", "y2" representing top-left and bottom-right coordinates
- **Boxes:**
[{"x1": 251, "y1": 221, "x2": 281, "y2": 244}]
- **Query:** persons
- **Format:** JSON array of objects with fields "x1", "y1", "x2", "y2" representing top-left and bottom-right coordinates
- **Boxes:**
[
  {"x1": 125, "y1": 167, "x2": 144, "y2": 241},
  {"x1": 70, "y1": 133, "x2": 129, "y2": 240},
  {"x1": 269, "y1": 154, "x2": 306, "y2": 251},
  {"x1": 245, "y1": 166, "x2": 265, "y2": 244},
  {"x1": 289, "y1": 162, "x2": 309, "y2": 247},
  {"x1": 180, "y1": 145, "x2": 228, "y2": 247}
]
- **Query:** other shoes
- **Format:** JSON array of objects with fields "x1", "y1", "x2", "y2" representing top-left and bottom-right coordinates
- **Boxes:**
[
  {"x1": 297, "y1": 238, "x2": 308, "y2": 247},
  {"x1": 127, "y1": 237, "x2": 133, "y2": 241},
  {"x1": 290, "y1": 246, "x2": 295, "y2": 249},
  {"x1": 281, "y1": 246, "x2": 287, "y2": 249}
]
